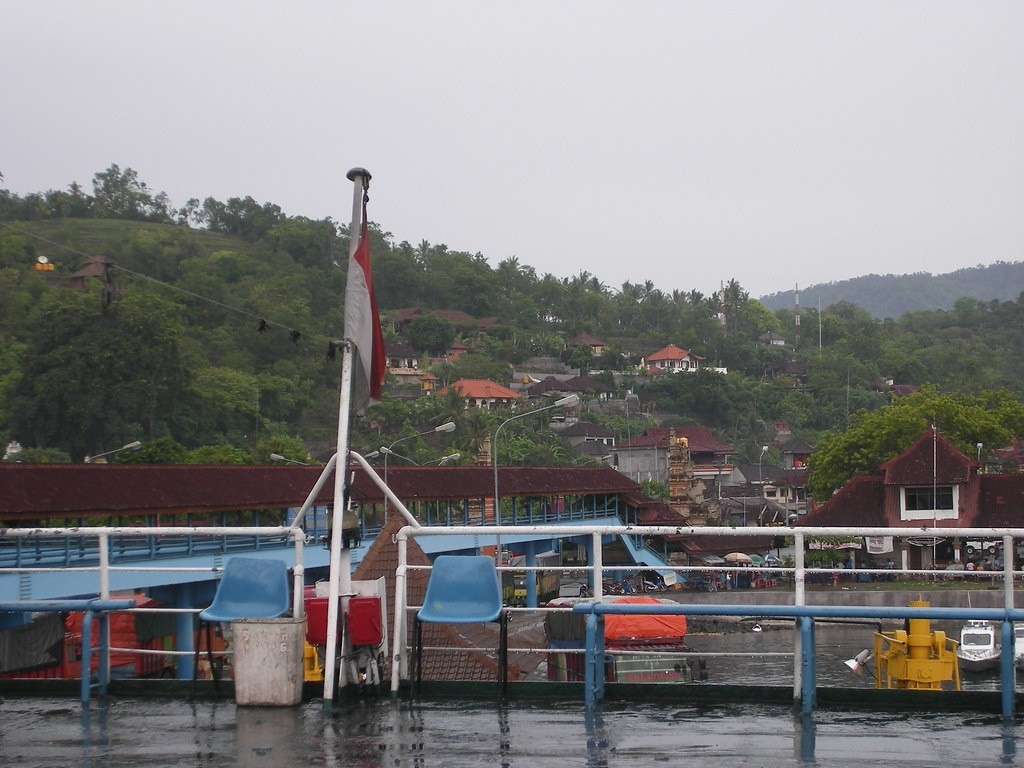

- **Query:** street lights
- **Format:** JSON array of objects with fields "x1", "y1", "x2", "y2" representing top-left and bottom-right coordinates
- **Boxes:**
[
  {"x1": 493, "y1": 393, "x2": 582, "y2": 586},
  {"x1": 759, "y1": 445, "x2": 769, "y2": 526},
  {"x1": 382, "y1": 420, "x2": 457, "y2": 524},
  {"x1": 379, "y1": 445, "x2": 460, "y2": 518}
]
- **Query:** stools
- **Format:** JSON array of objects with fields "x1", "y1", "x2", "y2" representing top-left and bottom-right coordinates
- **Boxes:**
[
  {"x1": 770, "y1": 579, "x2": 777, "y2": 588},
  {"x1": 765, "y1": 580, "x2": 771, "y2": 588},
  {"x1": 755, "y1": 579, "x2": 762, "y2": 589}
]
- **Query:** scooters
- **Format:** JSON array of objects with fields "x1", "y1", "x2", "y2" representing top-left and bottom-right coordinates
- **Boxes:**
[{"x1": 578, "y1": 575, "x2": 669, "y2": 597}]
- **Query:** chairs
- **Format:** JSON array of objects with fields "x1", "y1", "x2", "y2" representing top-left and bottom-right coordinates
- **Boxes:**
[
  {"x1": 407, "y1": 554, "x2": 510, "y2": 707},
  {"x1": 189, "y1": 558, "x2": 291, "y2": 702}
]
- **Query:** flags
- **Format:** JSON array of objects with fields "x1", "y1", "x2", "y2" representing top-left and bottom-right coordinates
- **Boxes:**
[{"x1": 345, "y1": 206, "x2": 385, "y2": 418}]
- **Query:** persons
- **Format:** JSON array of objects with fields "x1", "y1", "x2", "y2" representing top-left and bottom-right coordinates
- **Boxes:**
[{"x1": 707, "y1": 554, "x2": 1021, "y2": 590}]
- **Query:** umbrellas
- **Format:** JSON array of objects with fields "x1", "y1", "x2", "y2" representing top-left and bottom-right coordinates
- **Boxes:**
[{"x1": 725, "y1": 552, "x2": 783, "y2": 587}]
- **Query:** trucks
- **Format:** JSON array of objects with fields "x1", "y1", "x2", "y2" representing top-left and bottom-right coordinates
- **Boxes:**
[
  {"x1": 510, "y1": 549, "x2": 563, "y2": 609},
  {"x1": 64, "y1": 595, "x2": 231, "y2": 680},
  {"x1": 0, "y1": 610, "x2": 93, "y2": 679}
]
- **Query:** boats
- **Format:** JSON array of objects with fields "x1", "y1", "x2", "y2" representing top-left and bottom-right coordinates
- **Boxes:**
[
  {"x1": 544, "y1": 593, "x2": 709, "y2": 682},
  {"x1": 1014, "y1": 622, "x2": 1024, "y2": 671},
  {"x1": 957, "y1": 620, "x2": 1002, "y2": 673}
]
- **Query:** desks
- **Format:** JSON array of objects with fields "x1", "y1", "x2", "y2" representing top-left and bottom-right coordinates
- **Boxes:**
[{"x1": 760, "y1": 578, "x2": 766, "y2": 581}]
